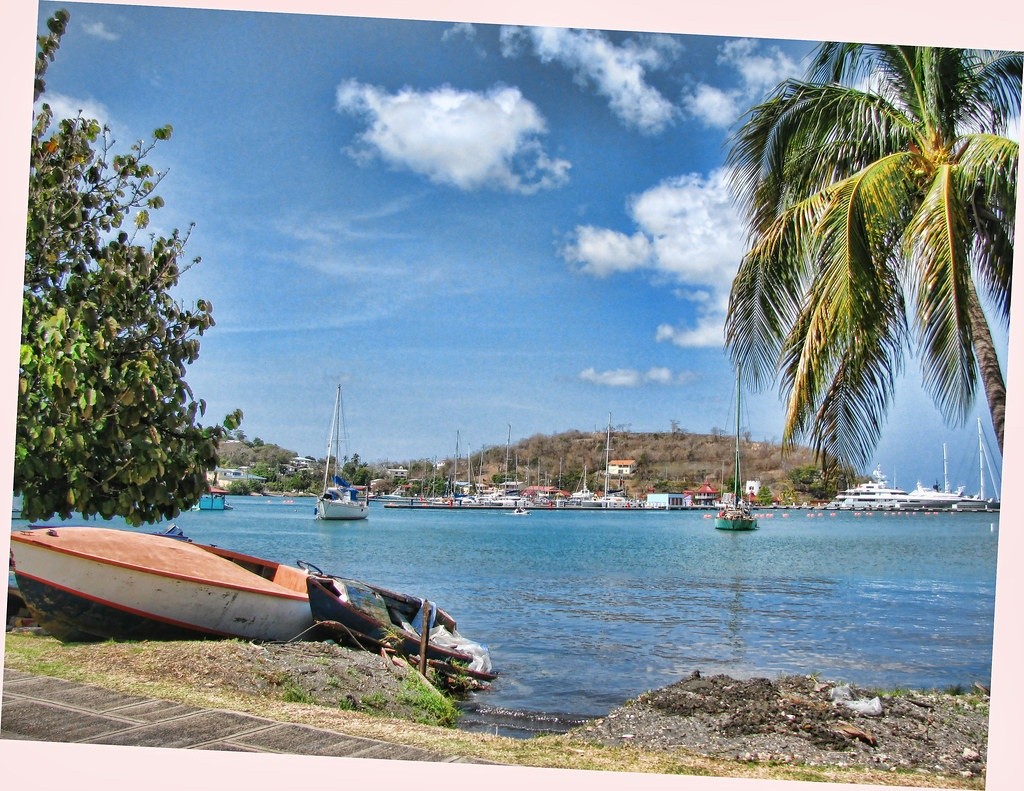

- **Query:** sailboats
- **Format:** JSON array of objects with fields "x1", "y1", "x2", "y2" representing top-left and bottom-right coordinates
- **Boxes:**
[
  {"x1": 392, "y1": 411, "x2": 637, "y2": 509},
  {"x1": 316, "y1": 383, "x2": 370, "y2": 520},
  {"x1": 713, "y1": 361, "x2": 759, "y2": 532},
  {"x1": 825, "y1": 417, "x2": 1000, "y2": 513}
]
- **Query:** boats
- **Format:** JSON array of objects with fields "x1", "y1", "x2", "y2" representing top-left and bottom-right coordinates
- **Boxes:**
[
  {"x1": 306, "y1": 570, "x2": 499, "y2": 691},
  {"x1": 10, "y1": 524, "x2": 327, "y2": 646}
]
